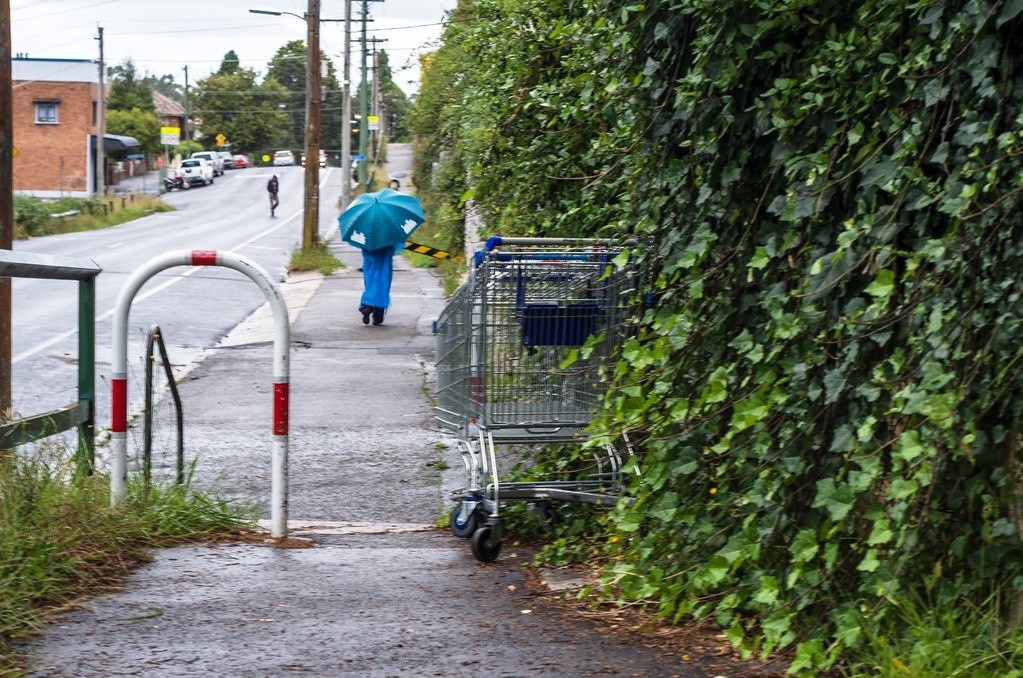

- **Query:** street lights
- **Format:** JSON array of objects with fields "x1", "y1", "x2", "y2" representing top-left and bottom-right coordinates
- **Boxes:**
[{"x1": 248, "y1": 0, "x2": 325, "y2": 252}]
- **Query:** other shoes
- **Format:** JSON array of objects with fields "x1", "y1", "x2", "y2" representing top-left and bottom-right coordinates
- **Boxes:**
[
  {"x1": 373, "y1": 320, "x2": 379, "y2": 325},
  {"x1": 363, "y1": 308, "x2": 370, "y2": 324}
]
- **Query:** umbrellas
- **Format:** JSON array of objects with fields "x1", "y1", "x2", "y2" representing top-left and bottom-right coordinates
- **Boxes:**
[{"x1": 338, "y1": 187, "x2": 426, "y2": 252}]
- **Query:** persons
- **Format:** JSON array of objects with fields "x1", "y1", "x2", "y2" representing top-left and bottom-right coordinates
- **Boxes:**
[
  {"x1": 358, "y1": 245, "x2": 395, "y2": 326},
  {"x1": 268, "y1": 175, "x2": 279, "y2": 217}
]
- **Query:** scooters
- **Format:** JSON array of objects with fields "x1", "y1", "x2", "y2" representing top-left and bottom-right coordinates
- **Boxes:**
[{"x1": 163, "y1": 170, "x2": 190, "y2": 192}]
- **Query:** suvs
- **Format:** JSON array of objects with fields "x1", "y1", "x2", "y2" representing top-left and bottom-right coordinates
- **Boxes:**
[
  {"x1": 179, "y1": 159, "x2": 229, "y2": 184},
  {"x1": 191, "y1": 152, "x2": 226, "y2": 176}
]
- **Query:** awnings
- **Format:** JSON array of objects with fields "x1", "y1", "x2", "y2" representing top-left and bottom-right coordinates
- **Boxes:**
[{"x1": 92, "y1": 135, "x2": 142, "y2": 151}]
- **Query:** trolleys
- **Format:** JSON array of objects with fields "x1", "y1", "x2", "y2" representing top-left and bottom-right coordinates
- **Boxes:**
[{"x1": 427, "y1": 234, "x2": 657, "y2": 563}]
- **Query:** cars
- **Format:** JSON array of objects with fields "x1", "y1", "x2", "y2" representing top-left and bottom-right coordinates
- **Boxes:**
[
  {"x1": 234, "y1": 155, "x2": 250, "y2": 168},
  {"x1": 302, "y1": 150, "x2": 328, "y2": 168},
  {"x1": 273, "y1": 151, "x2": 297, "y2": 166},
  {"x1": 217, "y1": 151, "x2": 235, "y2": 169}
]
